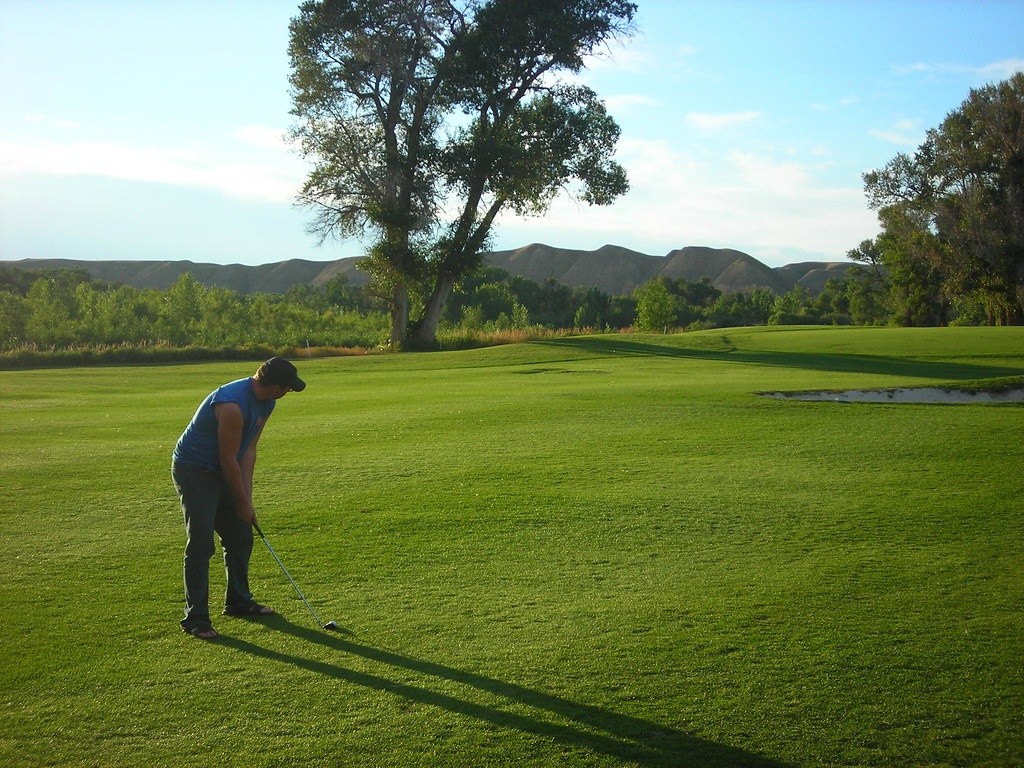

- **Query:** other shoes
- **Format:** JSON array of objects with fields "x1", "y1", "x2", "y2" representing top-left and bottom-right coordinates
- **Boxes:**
[
  {"x1": 189, "y1": 626, "x2": 220, "y2": 640},
  {"x1": 222, "y1": 603, "x2": 274, "y2": 615}
]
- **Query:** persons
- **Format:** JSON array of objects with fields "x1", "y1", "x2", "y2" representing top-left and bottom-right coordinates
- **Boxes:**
[{"x1": 171, "y1": 357, "x2": 307, "y2": 638}]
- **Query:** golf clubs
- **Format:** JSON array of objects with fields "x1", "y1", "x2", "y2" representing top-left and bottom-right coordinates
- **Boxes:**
[{"x1": 253, "y1": 520, "x2": 337, "y2": 630}]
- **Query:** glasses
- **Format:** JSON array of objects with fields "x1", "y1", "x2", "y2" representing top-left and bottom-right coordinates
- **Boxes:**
[{"x1": 281, "y1": 385, "x2": 293, "y2": 393}]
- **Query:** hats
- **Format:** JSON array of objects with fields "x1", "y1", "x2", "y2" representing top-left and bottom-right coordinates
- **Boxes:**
[{"x1": 266, "y1": 356, "x2": 306, "y2": 392}]
